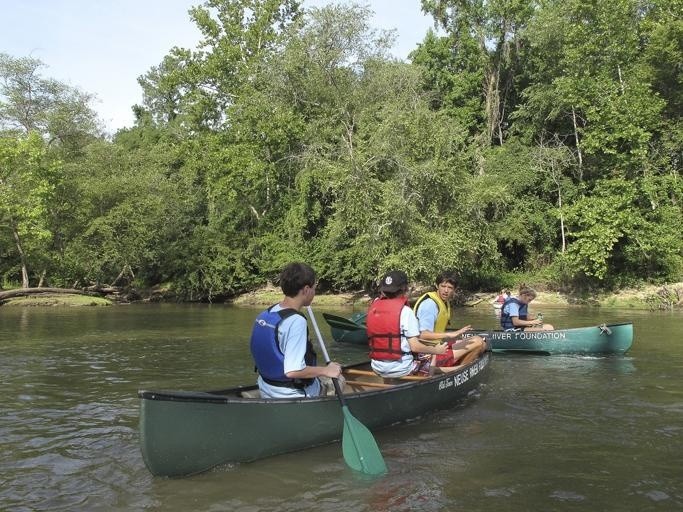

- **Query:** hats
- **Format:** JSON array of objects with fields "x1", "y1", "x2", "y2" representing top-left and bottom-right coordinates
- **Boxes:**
[{"x1": 378, "y1": 271, "x2": 407, "y2": 293}]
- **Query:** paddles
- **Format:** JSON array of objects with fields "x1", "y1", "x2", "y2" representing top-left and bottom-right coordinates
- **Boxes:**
[
  {"x1": 305, "y1": 302, "x2": 389, "y2": 473},
  {"x1": 322, "y1": 311, "x2": 464, "y2": 347}
]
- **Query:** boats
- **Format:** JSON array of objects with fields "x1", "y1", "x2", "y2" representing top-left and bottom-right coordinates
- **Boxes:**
[
  {"x1": 322, "y1": 312, "x2": 635, "y2": 353},
  {"x1": 138, "y1": 338, "x2": 490, "y2": 479}
]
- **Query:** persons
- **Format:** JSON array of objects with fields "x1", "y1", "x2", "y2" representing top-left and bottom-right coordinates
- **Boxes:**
[
  {"x1": 494, "y1": 291, "x2": 504, "y2": 302},
  {"x1": 413, "y1": 270, "x2": 488, "y2": 367},
  {"x1": 248, "y1": 261, "x2": 347, "y2": 399},
  {"x1": 499, "y1": 282, "x2": 554, "y2": 330},
  {"x1": 498, "y1": 287, "x2": 510, "y2": 300},
  {"x1": 364, "y1": 269, "x2": 449, "y2": 380}
]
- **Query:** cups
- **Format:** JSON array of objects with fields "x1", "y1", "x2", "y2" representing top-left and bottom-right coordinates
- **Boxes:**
[{"x1": 535, "y1": 313, "x2": 544, "y2": 330}]
